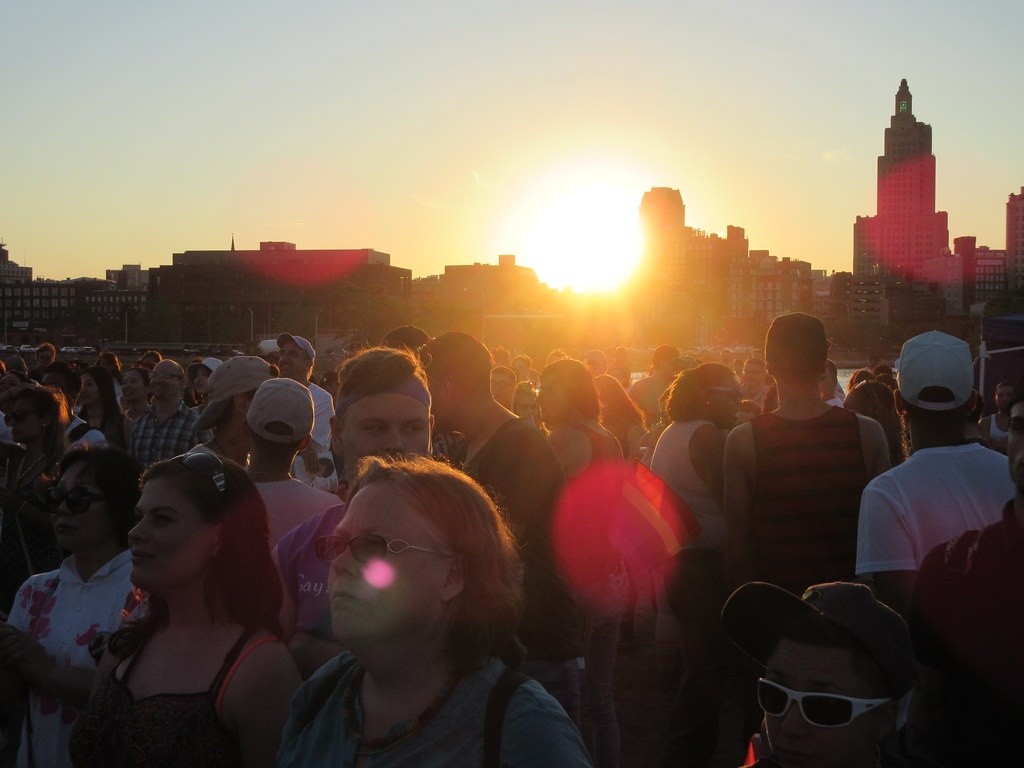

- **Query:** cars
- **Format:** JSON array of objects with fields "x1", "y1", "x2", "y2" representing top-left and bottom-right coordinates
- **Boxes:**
[{"x1": 3, "y1": 345, "x2": 93, "y2": 353}]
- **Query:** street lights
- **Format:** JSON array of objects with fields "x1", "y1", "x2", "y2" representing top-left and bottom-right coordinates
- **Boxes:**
[
  {"x1": 315, "y1": 311, "x2": 317, "y2": 337},
  {"x1": 125, "y1": 310, "x2": 128, "y2": 343},
  {"x1": 248, "y1": 307, "x2": 254, "y2": 352}
]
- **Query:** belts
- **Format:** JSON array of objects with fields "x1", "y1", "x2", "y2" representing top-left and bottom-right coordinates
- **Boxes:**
[{"x1": 676, "y1": 548, "x2": 722, "y2": 563}]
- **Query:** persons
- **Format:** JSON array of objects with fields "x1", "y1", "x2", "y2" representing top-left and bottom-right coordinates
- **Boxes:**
[
  {"x1": 0, "y1": 313, "x2": 1024, "y2": 768},
  {"x1": 720, "y1": 582, "x2": 915, "y2": 768},
  {"x1": 276, "y1": 451, "x2": 590, "y2": 768},
  {"x1": 0, "y1": 447, "x2": 158, "y2": 768},
  {"x1": 68, "y1": 443, "x2": 301, "y2": 767}
]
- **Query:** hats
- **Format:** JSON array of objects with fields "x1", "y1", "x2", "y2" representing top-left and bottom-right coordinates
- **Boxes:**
[
  {"x1": 247, "y1": 378, "x2": 315, "y2": 443},
  {"x1": 276, "y1": 332, "x2": 316, "y2": 359},
  {"x1": 187, "y1": 356, "x2": 223, "y2": 382},
  {"x1": 895, "y1": 330, "x2": 974, "y2": 412},
  {"x1": 190, "y1": 356, "x2": 279, "y2": 432},
  {"x1": 672, "y1": 356, "x2": 697, "y2": 370},
  {"x1": 381, "y1": 325, "x2": 433, "y2": 351},
  {"x1": 720, "y1": 581, "x2": 915, "y2": 701}
]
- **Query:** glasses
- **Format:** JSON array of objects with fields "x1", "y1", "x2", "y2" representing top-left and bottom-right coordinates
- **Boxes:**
[
  {"x1": 4, "y1": 410, "x2": 26, "y2": 425},
  {"x1": 708, "y1": 387, "x2": 744, "y2": 402},
  {"x1": 757, "y1": 678, "x2": 892, "y2": 728},
  {"x1": 1008, "y1": 417, "x2": 1024, "y2": 433},
  {"x1": 315, "y1": 534, "x2": 439, "y2": 564},
  {"x1": 44, "y1": 487, "x2": 109, "y2": 515},
  {"x1": 168, "y1": 453, "x2": 225, "y2": 496},
  {"x1": 490, "y1": 380, "x2": 513, "y2": 390}
]
